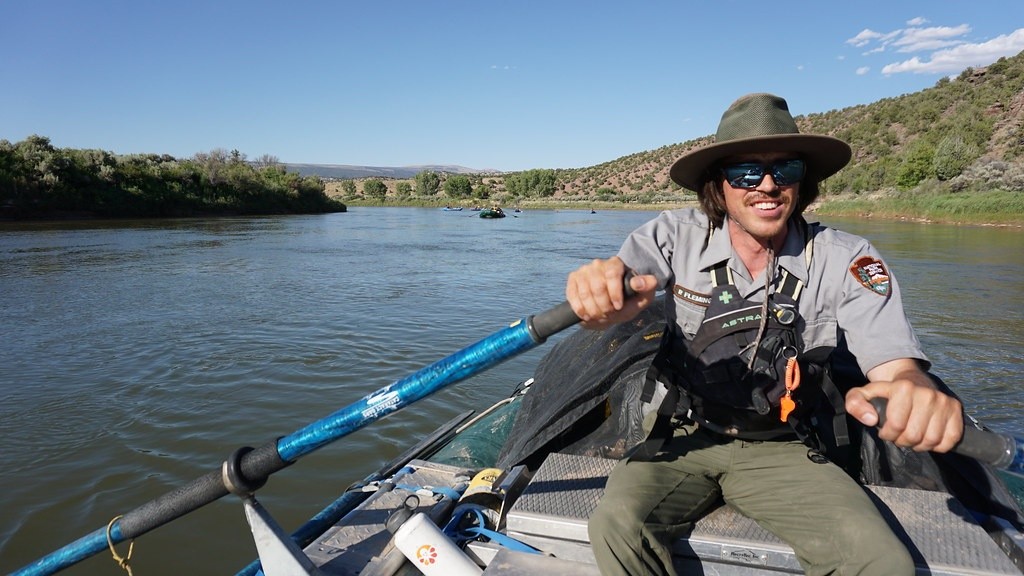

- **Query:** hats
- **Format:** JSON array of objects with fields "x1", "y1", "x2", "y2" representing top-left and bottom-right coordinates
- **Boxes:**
[{"x1": 669, "y1": 92, "x2": 854, "y2": 193}]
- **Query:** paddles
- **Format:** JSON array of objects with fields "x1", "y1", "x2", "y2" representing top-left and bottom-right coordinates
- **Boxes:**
[
  {"x1": 870, "y1": 398, "x2": 1024, "y2": 475},
  {"x1": 5, "y1": 271, "x2": 645, "y2": 576},
  {"x1": 502, "y1": 211, "x2": 518, "y2": 218},
  {"x1": 469, "y1": 212, "x2": 480, "y2": 217}
]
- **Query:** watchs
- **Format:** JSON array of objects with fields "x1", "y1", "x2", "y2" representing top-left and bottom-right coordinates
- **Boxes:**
[{"x1": 769, "y1": 299, "x2": 800, "y2": 326}]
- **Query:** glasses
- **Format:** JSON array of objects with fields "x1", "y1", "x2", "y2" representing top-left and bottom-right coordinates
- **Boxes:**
[{"x1": 719, "y1": 159, "x2": 807, "y2": 189}]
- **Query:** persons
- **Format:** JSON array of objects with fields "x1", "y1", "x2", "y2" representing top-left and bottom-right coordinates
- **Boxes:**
[
  {"x1": 566, "y1": 93, "x2": 963, "y2": 576},
  {"x1": 484, "y1": 204, "x2": 497, "y2": 210},
  {"x1": 446, "y1": 201, "x2": 453, "y2": 209}
]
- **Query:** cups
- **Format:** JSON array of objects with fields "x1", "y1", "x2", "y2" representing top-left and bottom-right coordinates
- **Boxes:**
[{"x1": 385, "y1": 495, "x2": 483, "y2": 576}]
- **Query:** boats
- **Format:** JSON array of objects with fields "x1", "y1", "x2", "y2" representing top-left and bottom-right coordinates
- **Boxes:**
[
  {"x1": 443, "y1": 207, "x2": 463, "y2": 212},
  {"x1": 479, "y1": 204, "x2": 506, "y2": 219}
]
